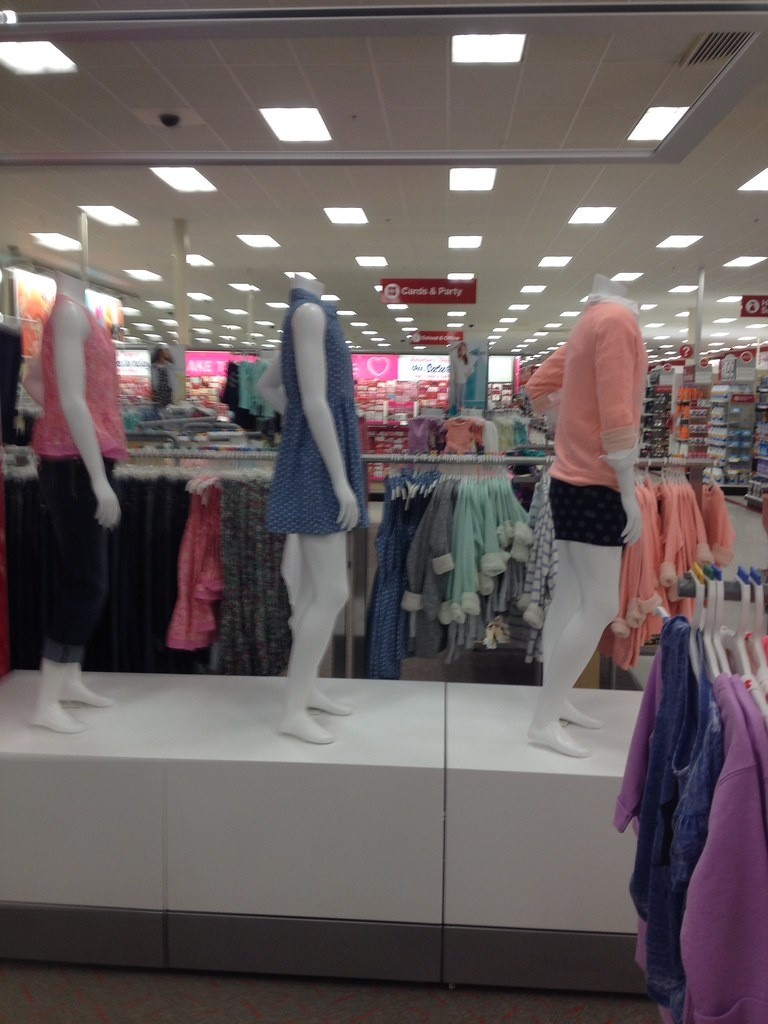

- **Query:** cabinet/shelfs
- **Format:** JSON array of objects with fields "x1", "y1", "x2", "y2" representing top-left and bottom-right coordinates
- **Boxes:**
[{"x1": 636, "y1": 351, "x2": 768, "y2": 511}]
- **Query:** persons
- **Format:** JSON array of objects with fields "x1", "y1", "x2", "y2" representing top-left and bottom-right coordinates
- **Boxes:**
[
  {"x1": 450, "y1": 342, "x2": 473, "y2": 411},
  {"x1": 150, "y1": 348, "x2": 178, "y2": 404},
  {"x1": 257, "y1": 273, "x2": 368, "y2": 743},
  {"x1": 525, "y1": 294, "x2": 648, "y2": 757},
  {"x1": 21, "y1": 276, "x2": 124, "y2": 732}
]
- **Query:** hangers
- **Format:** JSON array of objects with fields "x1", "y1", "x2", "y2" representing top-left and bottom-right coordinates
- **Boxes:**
[
  {"x1": 1, "y1": 431, "x2": 768, "y2": 727},
  {"x1": 415, "y1": 407, "x2": 525, "y2": 422}
]
